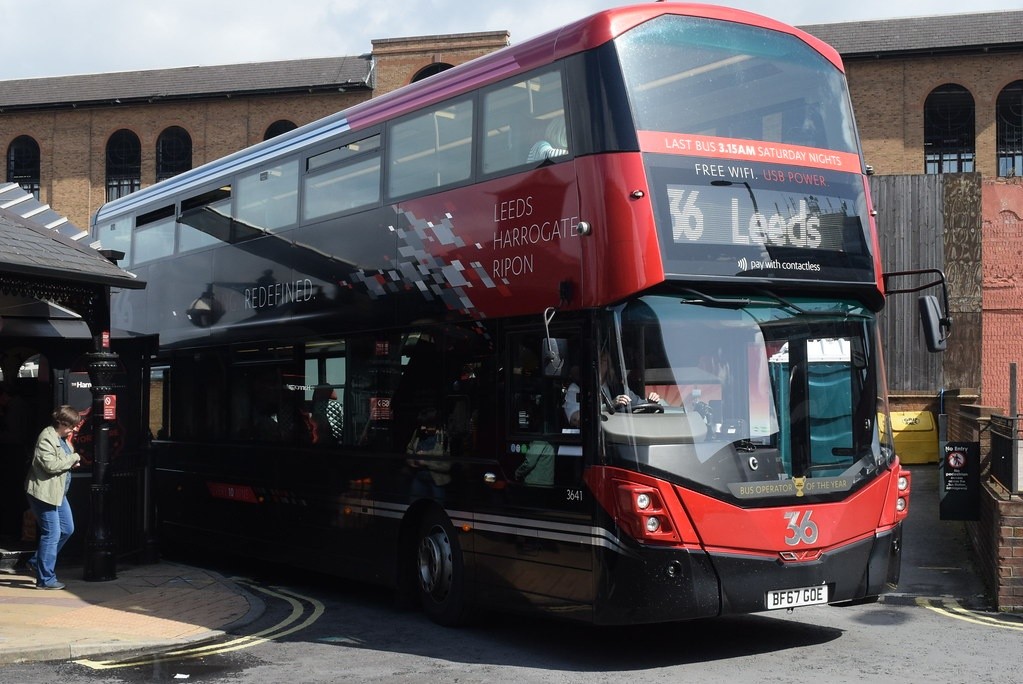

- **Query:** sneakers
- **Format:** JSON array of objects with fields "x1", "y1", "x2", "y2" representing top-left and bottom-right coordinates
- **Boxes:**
[
  {"x1": 36, "y1": 578, "x2": 66, "y2": 590},
  {"x1": 26, "y1": 561, "x2": 40, "y2": 576}
]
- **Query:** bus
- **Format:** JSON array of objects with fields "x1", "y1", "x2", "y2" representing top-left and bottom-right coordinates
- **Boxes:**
[{"x1": 88, "y1": 0, "x2": 954, "y2": 632}]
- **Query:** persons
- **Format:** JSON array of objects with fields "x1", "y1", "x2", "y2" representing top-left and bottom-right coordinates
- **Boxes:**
[
  {"x1": 402, "y1": 379, "x2": 555, "y2": 507},
  {"x1": 526, "y1": 116, "x2": 568, "y2": 164},
  {"x1": 562, "y1": 345, "x2": 660, "y2": 430},
  {"x1": 25, "y1": 404, "x2": 82, "y2": 589}
]
